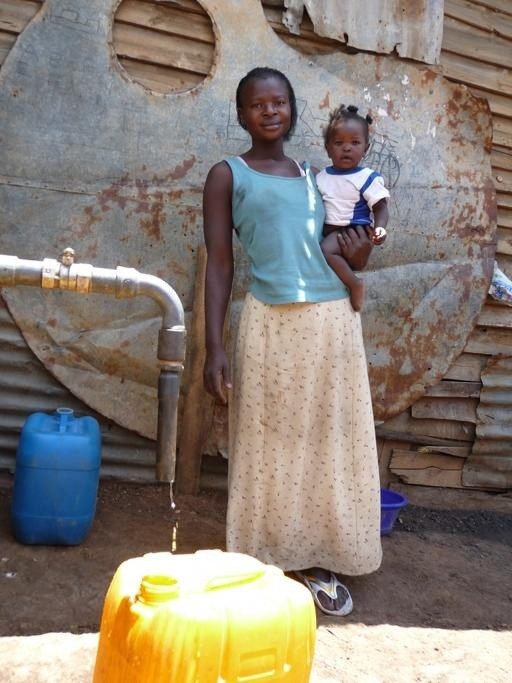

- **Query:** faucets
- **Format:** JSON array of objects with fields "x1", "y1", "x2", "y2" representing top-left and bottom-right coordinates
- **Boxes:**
[{"x1": 1, "y1": 252, "x2": 189, "y2": 483}]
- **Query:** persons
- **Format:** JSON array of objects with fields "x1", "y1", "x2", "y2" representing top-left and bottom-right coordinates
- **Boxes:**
[
  {"x1": 310, "y1": 105, "x2": 390, "y2": 311},
  {"x1": 204, "y1": 66, "x2": 384, "y2": 616}
]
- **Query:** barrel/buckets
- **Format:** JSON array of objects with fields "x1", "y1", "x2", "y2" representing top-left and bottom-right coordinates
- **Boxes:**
[
  {"x1": 12, "y1": 406, "x2": 101, "y2": 545},
  {"x1": 92, "y1": 548, "x2": 317, "y2": 683}
]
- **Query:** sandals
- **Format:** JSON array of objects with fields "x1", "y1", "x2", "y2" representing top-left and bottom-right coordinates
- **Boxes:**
[{"x1": 293, "y1": 567, "x2": 354, "y2": 617}]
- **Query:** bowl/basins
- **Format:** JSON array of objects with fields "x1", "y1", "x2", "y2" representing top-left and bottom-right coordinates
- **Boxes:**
[{"x1": 380, "y1": 489, "x2": 406, "y2": 538}]
